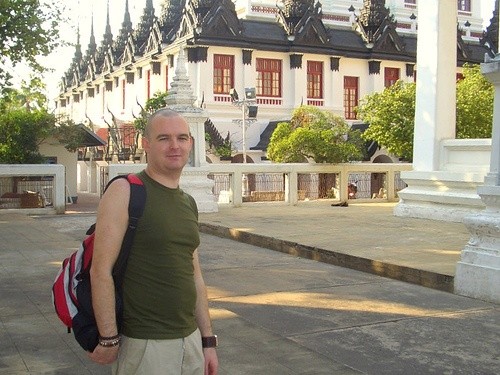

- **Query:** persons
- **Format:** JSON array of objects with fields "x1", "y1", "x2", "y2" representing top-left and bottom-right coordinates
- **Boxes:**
[{"x1": 84, "y1": 108, "x2": 220, "y2": 375}]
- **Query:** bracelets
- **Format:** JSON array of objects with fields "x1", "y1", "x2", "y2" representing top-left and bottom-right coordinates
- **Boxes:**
[
  {"x1": 98, "y1": 335, "x2": 121, "y2": 347},
  {"x1": 202, "y1": 334, "x2": 218, "y2": 348}
]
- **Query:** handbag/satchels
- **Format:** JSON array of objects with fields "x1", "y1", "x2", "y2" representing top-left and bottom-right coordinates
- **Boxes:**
[{"x1": 49, "y1": 175, "x2": 146, "y2": 354}]
